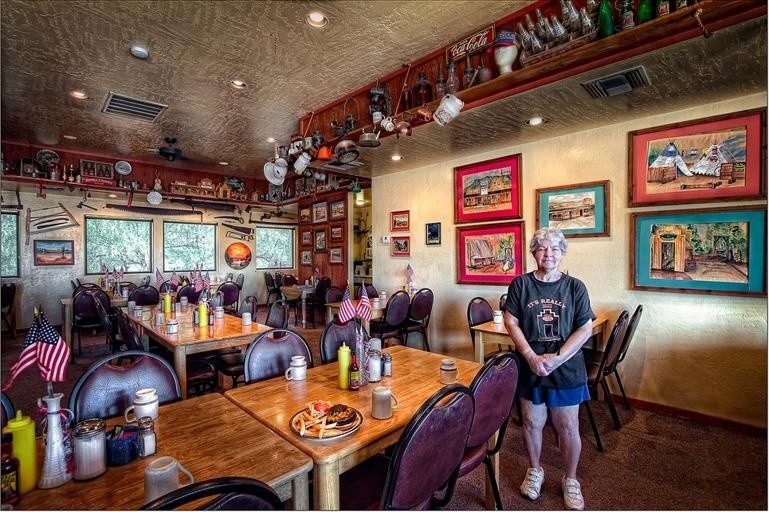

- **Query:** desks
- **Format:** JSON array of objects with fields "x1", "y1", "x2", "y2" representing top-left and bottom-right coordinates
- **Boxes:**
[
  {"x1": 1, "y1": 392, "x2": 315, "y2": 511},
  {"x1": 222, "y1": 342, "x2": 507, "y2": 509},
  {"x1": 321, "y1": 297, "x2": 403, "y2": 341},
  {"x1": 470, "y1": 315, "x2": 611, "y2": 372}
]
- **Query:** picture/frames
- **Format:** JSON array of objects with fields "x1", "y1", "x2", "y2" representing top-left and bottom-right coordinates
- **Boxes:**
[
  {"x1": 390, "y1": 210, "x2": 441, "y2": 257},
  {"x1": 629, "y1": 204, "x2": 767, "y2": 298},
  {"x1": 298, "y1": 199, "x2": 347, "y2": 266},
  {"x1": 535, "y1": 179, "x2": 611, "y2": 239},
  {"x1": 454, "y1": 153, "x2": 523, "y2": 224},
  {"x1": 456, "y1": 220, "x2": 527, "y2": 285},
  {"x1": 33, "y1": 240, "x2": 74, "y2": 266},
  {"x1": 627, "y1": 106, "x2": 768, "y2": 208}
]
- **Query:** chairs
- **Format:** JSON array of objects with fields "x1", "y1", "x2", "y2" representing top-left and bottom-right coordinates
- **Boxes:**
[
  {"x1": 465, "y1": 295, "x2": 504, "y2": 368},
  {"x1": 324, "y1": 285, "x2": 345, "y2": 303},
  {"x1": 343, "y1": 383, "x2": 477, "y2": 510},
  {"x1": 407, "y1": 286, "x2": 436, "y2": 353},
  {"x1": 62, "y1": 348, "x2": 186, "y2": 422},
  {"x1": 124, "y1": 475, "x2": 287, "y2": 512},
  {"x1": 5, "y1": 272, "x2": 294, "y2": 399},
  {"x1": 369, "y1": 289, "x2": 411, "y2": 349},
  {"x1": 356, "y1": 283, "x2": 380, "y2": 300},
  {"x1": 261, "y1": 272, "x2": 335, "y2": 330},
  {"x1": 242, "y1": 327, "x2": 316, "y2": 384},
  {"x1": 424, "y1": 350, "x2": 525, "y2": 510},
  {"x1": 553, "y1": 309, "x2": 632, "y2": 451},
  {"x1": 320, "y1": 315, "x2": 372, "y2": 363},
  {"x1": 582, "y1": 301, "x2": 642, "y2": 432}
]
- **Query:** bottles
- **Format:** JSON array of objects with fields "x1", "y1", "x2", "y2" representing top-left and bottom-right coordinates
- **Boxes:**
[
  {"x1": 0, "y1": 408, "x2": 42, "y2": 497},
  {"x1": 159, "y1": 293, "x2": 218, "y2": 327},
  {"x1": 1, "y1": 432, "x2": 21, "y2": 507},
  {"x1": 251, "y1": 192, "x2": 258, "y2": 201},
  {"x1": 337, "y1": 334, "x2": 392, "y2": 390},
  {"x1": 208, "y1": 325, "x2": 214, "y2": 338},
  {"x1": 277, "y1": 141, "x2": 303, "y2": 158},
  {"x1": 69, "y1": 418, "x2": 107, "y2": 483},
  {"x1": 50, "y1": 163, "x2": 110, "y2": 183},
  {"x1": 399, "y1": 1, "x2": 698, "y2": 111},
  {"x1": 200, "y1": 182, "x2": 207, "y2": 195},
  {"x1": 136, "y1": 416, "x2": 158, "y2": 455},
  {"x1": 402, "y1": 285, "x2": 415, "y2": 304},
  {"x1": 38, "y1": 393, "x2": 76, "y2": 490},
  {"x1": 114, "y1": 281, "x2": 129, "y2": 298},
  {"x1": 117, "y1": 174, "x2": 124, "y2": 188}
]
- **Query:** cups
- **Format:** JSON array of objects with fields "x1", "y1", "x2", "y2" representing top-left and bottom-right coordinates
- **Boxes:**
[
  {"x1": 104, "y1": 424, "x2": 142, "y2": 467},
  {"x1": 124, "y1": 386, "x2": 158, "y2": 423},
  {"x1": 372, "y1": 94, "x2": 465, "y2": 136},
  {"x1": 493, "y1": 310, "x2": 504, "y2": 324},
  {"x1": 438, "y1": 359, "x2": 458, "y2": 384},
  {"x1": 372, "y1": 386, "x2": 399, "y2": 420},
  {"x1": 285, "y1": 355, "x2": 308, "y2": 381},
  {"x1": 372, "y1": 291, "x2": 387, "y2": 307},
  {"x1": 144, "y1": 456, "x2": 194, "y2": 502},
  {"x1": 215, "y1": 305, "x2": 225, "y2": 319},
  {"x1": 335, "y1": 117, "x2": 357, "y2": 136},
  {"x1": 125, "y1": 300, "x2": 180, "y2": 335},
  {"x1": 305, "y1": 280, "x2": 309, "y2": 286},
  {"x1": 303, "y1": 169, "x2": 327, "y2": 181},
  {"x1": 302, "y1": 134, "x2": 322, "y2": 150},
  {"x1": 241, "y1": 312, "x2": 253, "y2": 326}
]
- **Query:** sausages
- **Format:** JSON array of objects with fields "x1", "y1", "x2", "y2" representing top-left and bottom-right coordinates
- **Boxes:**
[{"x1": 338, "y1": 410, "x2": 356, "y2": 424}]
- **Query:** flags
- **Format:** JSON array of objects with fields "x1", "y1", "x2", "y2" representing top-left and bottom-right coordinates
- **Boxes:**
[
  {"x1": 353, "y1": 282, "x2": 371, "y2": 322},
  {"x1": 405, "y1": 278, "x2": 411, "y2": 283},
  {"x1": 37, "y1": 311, "x2": 71, "y2": 383},
  {"x1": 1, "y1": 315, "x2": 39, "y2": 396},
  {"x1": 336, "y1": 282, "x2": 356, "y2": 323},
  {"x1": 405, "y1": 266, "x2": 414, "y2": 277},
  {"x1": 101, "y1": 264, "x2": 210, "y2": 292}
]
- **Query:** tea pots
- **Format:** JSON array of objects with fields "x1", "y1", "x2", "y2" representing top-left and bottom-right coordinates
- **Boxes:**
[
  {"x1": 333, "y1": 139, "x2": 360, "y2": 164},
  {"x1": 316, "y1": 145, "x2": 334, "y2": 161},
  {"x1": 359, "y1": 130, "x2": 382, "y2": 147}
]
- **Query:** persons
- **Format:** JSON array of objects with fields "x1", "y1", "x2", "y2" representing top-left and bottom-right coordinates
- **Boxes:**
[{"x1": 502, "y1": 226, "x2": 597, "y2": 510}]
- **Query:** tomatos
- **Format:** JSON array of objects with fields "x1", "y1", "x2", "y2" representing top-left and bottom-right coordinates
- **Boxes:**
[{"x1": 313, "y1": 401, "x2": 328, "y2": 412}]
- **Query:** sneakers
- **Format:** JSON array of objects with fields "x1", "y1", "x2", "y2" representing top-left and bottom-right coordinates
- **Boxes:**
[
  {"x1": 520, "y1": 466, "x2": 545, "y2": 501},
  {"x1": 561, "y1": 475, "x2": 585, "y2": 510}
]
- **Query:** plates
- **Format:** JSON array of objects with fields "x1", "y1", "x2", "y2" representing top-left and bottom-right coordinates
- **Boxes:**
[
  {"x1": 36, "y1": 150, "x2": 60, "y2": 168},
  {"x1": 15, "y1": 158, "x2": 43, "y2": 178},
  {"x1": 290, "y1": 404, "x2": 365, "y2": 442}
]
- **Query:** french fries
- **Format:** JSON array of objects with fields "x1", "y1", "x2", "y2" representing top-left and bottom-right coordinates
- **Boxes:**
[{"x1": 297, "y1": 401, "x2": 341, "y2": 439}]
